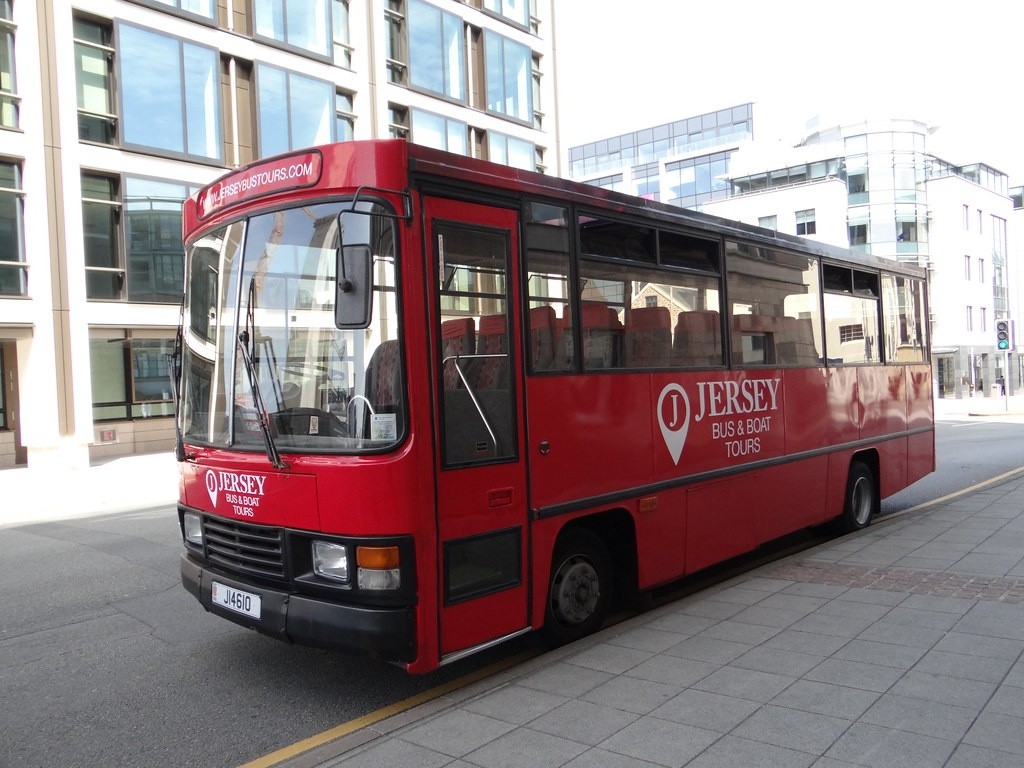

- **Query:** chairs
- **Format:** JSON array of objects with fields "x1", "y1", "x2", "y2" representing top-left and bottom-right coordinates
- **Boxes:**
[{"x1": 363, "y1": 300, "x2": 819, "y2": 446}]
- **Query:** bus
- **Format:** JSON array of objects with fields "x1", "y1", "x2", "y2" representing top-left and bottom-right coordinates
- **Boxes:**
[{"x1": 173, "y1": 139, "x2": 936, "y2": 678}]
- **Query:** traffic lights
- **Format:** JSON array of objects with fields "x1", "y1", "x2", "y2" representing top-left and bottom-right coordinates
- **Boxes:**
[{"x1": 995, "y1": 319, "x2": 1015, "y2": 351}]
